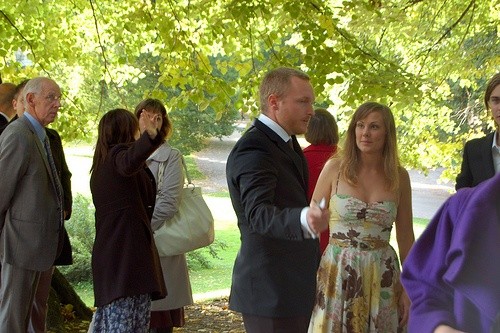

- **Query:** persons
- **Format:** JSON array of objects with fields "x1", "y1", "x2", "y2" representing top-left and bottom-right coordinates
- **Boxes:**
[
  {"x1": 135, "y1": 98, "x2": 193, "y2": 333},
  {"x1": 226, "y1": 67, "x2": 331, "y2": 333},
  {"x1": 0, "y1": 77, "x2": 63, "y2": 333},
  {"x1": 0, "y1": 83, "x2": 73, "y2": 265},
  {"x1": 455, "y1": 73, "x2": 500, "y2": 190},
  {"x1": 400, "y1": 171, "x2": 500, "y2": 333},
  {"x1": 89, "y1": 108, "x2": 167, "y2": 333},
  {"x1": 307, "y1": 102, "x2": 415, "y2": 333},
  {"x1": 303, "y1": 110, "x2": 339, "y2": 253}
]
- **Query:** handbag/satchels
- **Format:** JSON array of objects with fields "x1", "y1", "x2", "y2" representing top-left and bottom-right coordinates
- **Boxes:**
[{"x1": 154, "y1": 149, "x2": 215, "y2": 257}]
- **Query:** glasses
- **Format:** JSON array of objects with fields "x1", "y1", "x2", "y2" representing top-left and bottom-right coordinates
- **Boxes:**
[{"x1": 43, "y1": 93, "x2": 62, "y2": 102}]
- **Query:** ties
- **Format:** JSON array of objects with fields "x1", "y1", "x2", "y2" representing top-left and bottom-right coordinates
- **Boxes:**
[
  {"x1": 44, "y1": 137, "x2": 64, "y2": 226},
  {"x1": 287, "y1": 137, "x2": 295, "y2": 151}
]
